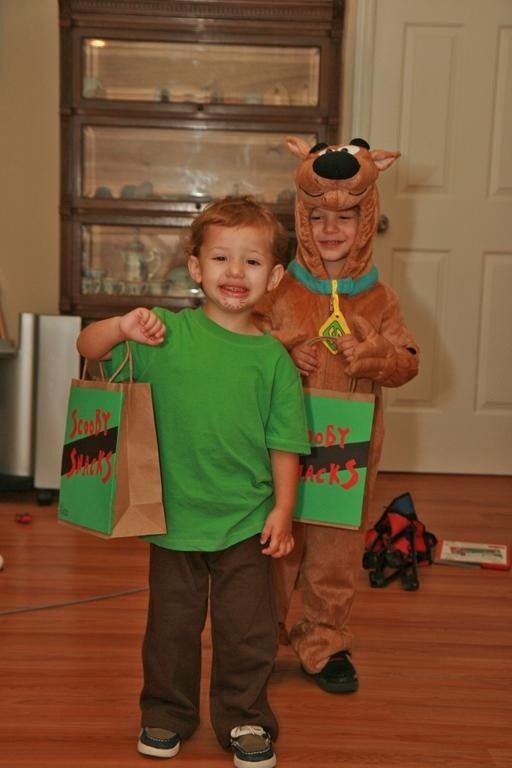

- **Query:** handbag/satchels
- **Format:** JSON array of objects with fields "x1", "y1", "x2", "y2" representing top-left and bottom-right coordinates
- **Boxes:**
[
  {"x1": 293, "y1": 336, "x2": 376, "y2": 530},
  {"x1": 58, "y1": 340, "x2": 167, "y2": 540},
  {"x1": 366, "y1": 492, "x2": 431, "y2": 567}
]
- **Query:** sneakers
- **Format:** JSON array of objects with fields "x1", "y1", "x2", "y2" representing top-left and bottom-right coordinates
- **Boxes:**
[
  {"x1": 226, "y1": 725, "x2": 277, "y2": 768},
  {"x1": 301, "y1": 651, "x2": 358, "y2": 694},
  {"x1": 136, "y1": 727, "x2": 181, "y2": 758}
]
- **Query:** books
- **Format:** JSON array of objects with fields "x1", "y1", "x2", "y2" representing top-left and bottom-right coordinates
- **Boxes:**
[{"x1": 432, "y1": 538, "x2": 512, "y2": 571}]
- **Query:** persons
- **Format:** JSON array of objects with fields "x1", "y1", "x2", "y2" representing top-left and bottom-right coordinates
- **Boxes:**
[
  {"x1": 250, "y1": 136, "x2": 421, "y2": 695},
  {"x1": 76, "y1": 194, "x2": 312, "y2": 768}
]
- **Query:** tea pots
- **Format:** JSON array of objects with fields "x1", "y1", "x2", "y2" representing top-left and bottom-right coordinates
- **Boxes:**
[{"x1": 113, "y1": 239, "x2": 163, "y2": 281}]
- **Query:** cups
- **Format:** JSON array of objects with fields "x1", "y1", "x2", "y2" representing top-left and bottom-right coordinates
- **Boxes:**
[{"x1": 81, "y1": 277, "x2": 167, "y2": 298}]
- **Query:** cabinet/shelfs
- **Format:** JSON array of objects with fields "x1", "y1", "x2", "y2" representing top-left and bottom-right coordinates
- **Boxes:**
[{"x1": 59, "y1": 0, "x2": 345, "y2": 380}]
